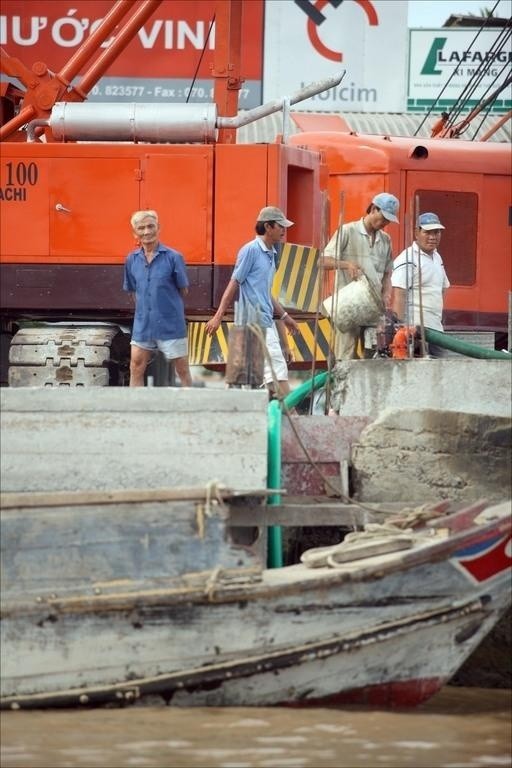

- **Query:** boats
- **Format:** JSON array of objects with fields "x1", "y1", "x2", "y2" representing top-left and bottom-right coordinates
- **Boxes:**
[{"x1": 0, "y1": 490, "x2": 512, "y2": 714}]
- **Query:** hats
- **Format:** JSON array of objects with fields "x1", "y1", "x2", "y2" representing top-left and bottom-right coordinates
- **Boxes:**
[
  {"x1": 416, "y1": 214, "x2": 446, "y2": 231},
  {"x1": 373, "y1": 193, "x2": 399, "y2": 224},
  {"x1": 257, "y1": 206, "x2": 294, "y2": 227}
]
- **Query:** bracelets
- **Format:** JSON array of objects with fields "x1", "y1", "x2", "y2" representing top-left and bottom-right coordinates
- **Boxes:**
[{"x1": 280, "y1": 312, "x2": 288, "y2": 321}]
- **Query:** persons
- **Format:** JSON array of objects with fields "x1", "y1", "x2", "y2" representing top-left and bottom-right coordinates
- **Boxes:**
[
  {"x1": 122, "y1": 209, "x2": 192, "y2": 387},
  {"x1": 317, "y1": 190, "x2": 400, "y2": 362},
  {"x1": 390, "y1": 212, "x2": 451, "y2": 357},
  {"x1": 205, "y1": 207, "x2": 301, "y2": 416}
]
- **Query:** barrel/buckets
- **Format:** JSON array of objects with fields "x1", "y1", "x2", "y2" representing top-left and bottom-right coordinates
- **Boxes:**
[{"x1": 322, "y1": 273, "x2": 387, "y2": 335}]
